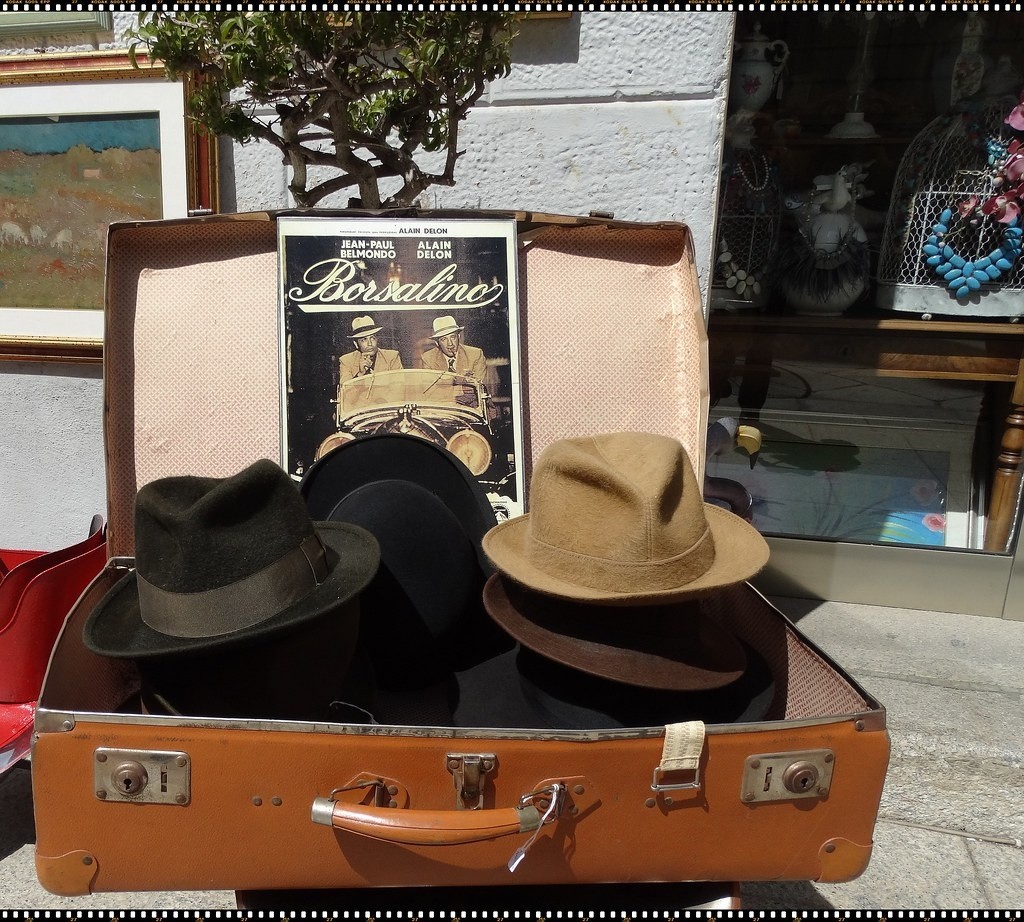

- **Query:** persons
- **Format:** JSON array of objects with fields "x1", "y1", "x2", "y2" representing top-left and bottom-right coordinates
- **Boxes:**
[
  {"x1": 339, "y1": 316, "x2": 404, "y2": 391},
  {"x1": 707, "y1": 205, "x2": 797, "y2": 450},
  {"x1": 420, "y1": 316, "x2": 502, "y2": 400}
]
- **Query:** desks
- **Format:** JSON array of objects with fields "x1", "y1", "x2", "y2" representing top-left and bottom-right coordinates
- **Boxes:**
[{"x1": 708, "y1": 311, "x2": 1024, "y2": 551}]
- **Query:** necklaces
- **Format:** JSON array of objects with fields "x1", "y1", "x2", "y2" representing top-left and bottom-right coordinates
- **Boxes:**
[
  {"x1": 777, "y1": 182, "x2": 871, "y2": 303},
  {"x1": 922, "y1": 168, "x2": 1024, "y2": 299},
  {"x1": 724, "y1": 138, "x2": 775, "y2": 198},
  {"x1": 716, "y1": 182, "x2": 782, "y2": 300}
]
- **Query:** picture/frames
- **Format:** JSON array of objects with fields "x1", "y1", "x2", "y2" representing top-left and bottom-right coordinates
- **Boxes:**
[{"x1": 0, "y1": 50, "x2": 223, "y2": 362}]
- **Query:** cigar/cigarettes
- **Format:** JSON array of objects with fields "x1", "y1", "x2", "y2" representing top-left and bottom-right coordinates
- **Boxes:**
[
  {"x1": 450, "y1": 348, "x2": 457, "y2": 355},
  {"x1": 363, "y1": 355, "x2": 367, "y2": 359}
]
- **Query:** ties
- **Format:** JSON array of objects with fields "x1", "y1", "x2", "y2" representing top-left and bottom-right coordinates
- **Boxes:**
[{"x1": 448, "y1": 359, "x2": 455, "y2": 372}]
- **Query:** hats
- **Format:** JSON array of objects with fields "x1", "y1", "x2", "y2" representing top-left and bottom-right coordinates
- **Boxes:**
[
  {"x1": 519, "y1": 637, "x2": 776, "y2": 729},
  {"x1": 83, "y1": 461, "x2": 381, "y2": 659},
  {"x1": 137, "y1": 595, "x2": 361, "y2": 714},
  {"x1": 347, "y1": 316, "x2": 383, "y2": 338},
  {"x1": 483, "y1": 571, "x2": 747, "y2": 690},
  {"x1": 429, "y1": 316, "x2": 465, "y2": 340},
  {"x1": 114, "y1": 690, "x2": 384, "y2": 724},
  {"x1": 482, "y1": 433, "x2": 770, "y2": 608},
  {"x1": 299, "y1": 433, "x2": 516, "y2": 691}
]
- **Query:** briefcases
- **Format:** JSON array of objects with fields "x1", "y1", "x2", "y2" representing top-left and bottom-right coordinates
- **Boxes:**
[{"x1": 31, "y1": 206, "x2": 891, "y2": 897}]
